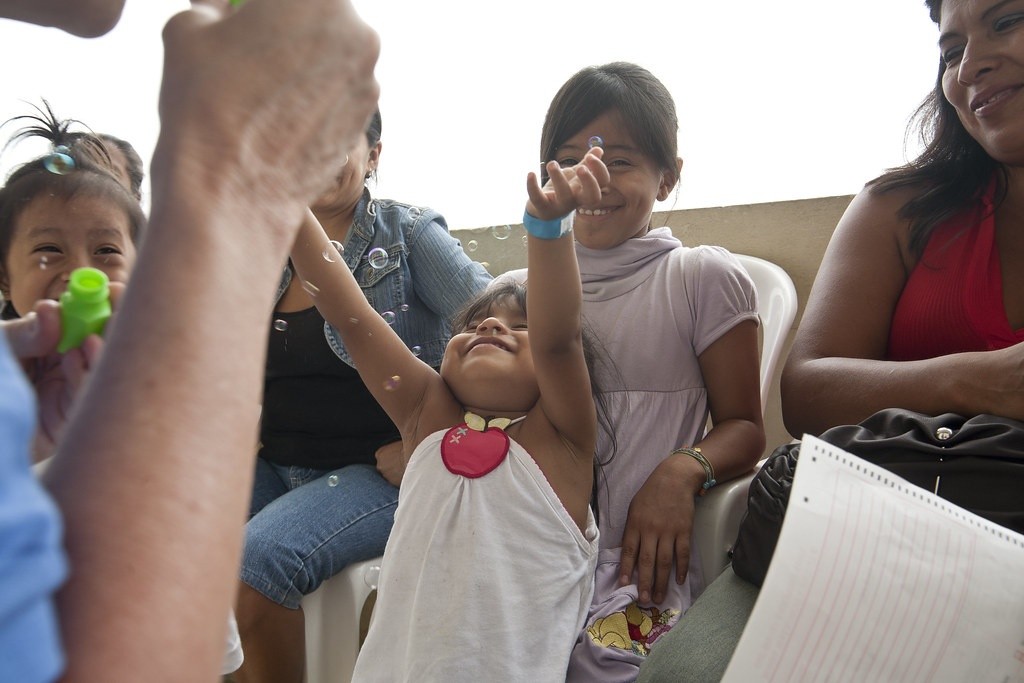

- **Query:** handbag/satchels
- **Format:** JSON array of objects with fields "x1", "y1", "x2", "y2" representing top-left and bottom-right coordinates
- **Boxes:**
[{"x1": 728, "y1": 407, "x2": 1024, "y2": 591}]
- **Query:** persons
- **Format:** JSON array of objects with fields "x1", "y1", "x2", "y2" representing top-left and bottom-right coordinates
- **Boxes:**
[
  {"x1": 633, "y1": 0, "x2": 1023, "y2": 683},
  {"x1": 289, "y1": 147, "x2": 610, "y2": 683},
  {"x1": 0, "y1": 102, "x2": 245, "y2": 683},
  {"x1": 235, "y1": 103, "x2": 494, "y2": 683},
  {"x1": 479, "y1": 63, "x2": 766, "y2": 683},
  {"x1": 0, "y1": 0, "x2": 382, "y2": 683}
]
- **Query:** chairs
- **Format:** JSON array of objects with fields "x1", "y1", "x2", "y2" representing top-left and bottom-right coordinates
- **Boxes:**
[
  {"x1": 691, "y1": 252, "x2": 799, "y2": 608},
  {"x1": 299, "y1": 556, "x2": 381, "y2": 683}
]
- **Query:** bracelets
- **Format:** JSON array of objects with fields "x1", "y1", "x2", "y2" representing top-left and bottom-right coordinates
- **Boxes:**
[
  {"x1": 523, "y1": 208, "x2": 576, "y2": 239},
  {"x1": 672, "y1": 446, "x2": 716, "y2": 498}
]
